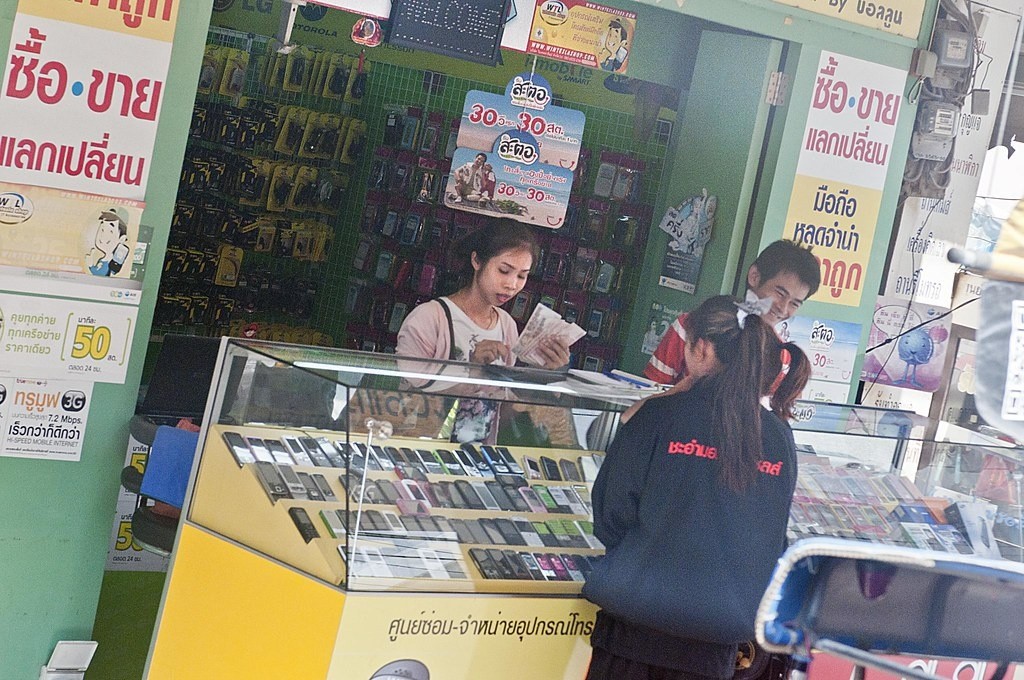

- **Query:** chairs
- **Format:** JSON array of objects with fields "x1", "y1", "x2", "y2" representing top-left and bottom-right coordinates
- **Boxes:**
[{"x1": 131, "y1": 424, "x2": 200, "y2": 560}]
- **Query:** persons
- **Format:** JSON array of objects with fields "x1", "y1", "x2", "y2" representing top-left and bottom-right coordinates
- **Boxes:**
[
  {"x1": 395, "y1": 216, "x2": 571, "y2": 393},
  {"x1": 643, "y1": 239, "x2": 822, "y2": 420},
  {"x1": 454, "y1": 152, "x2": 490, "y2": 207},
  {"x1": 580, "y1": 292, "x2": 812, "y2": 680}
]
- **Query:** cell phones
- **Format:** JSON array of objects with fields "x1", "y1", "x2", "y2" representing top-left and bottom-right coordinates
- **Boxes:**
[
  {"x1": 783, "y1": 469, "x2": 900, "y2": 541},
  {"x1": 222, "y1": 432, "x2": 607, "y2": 583}
]
